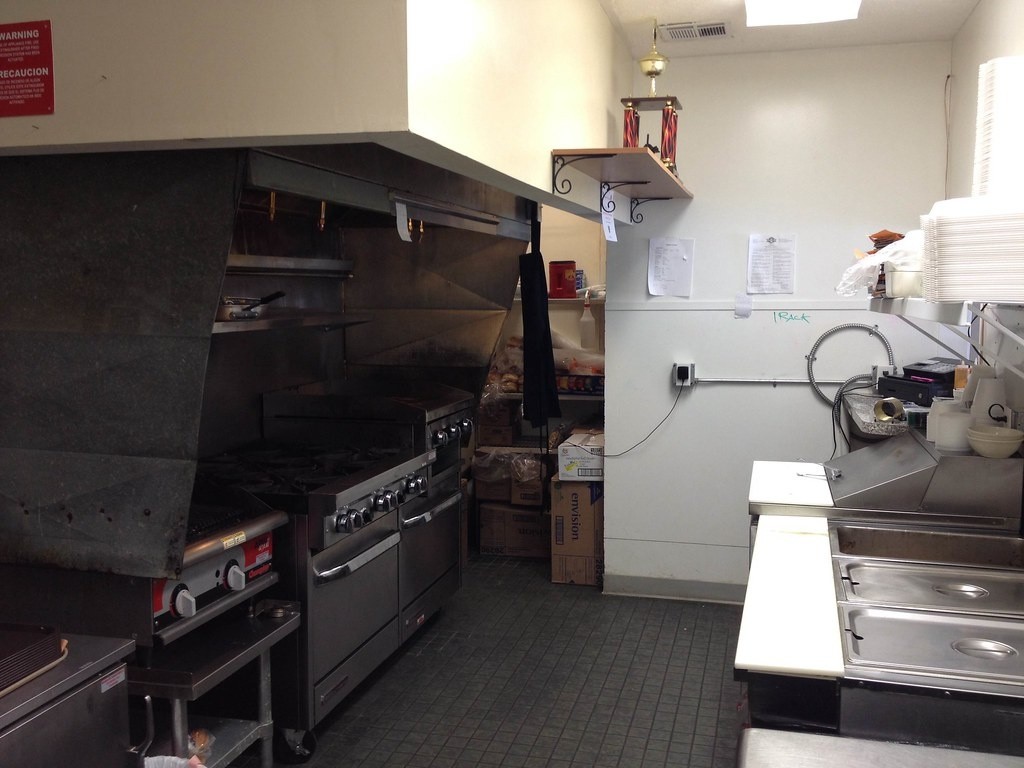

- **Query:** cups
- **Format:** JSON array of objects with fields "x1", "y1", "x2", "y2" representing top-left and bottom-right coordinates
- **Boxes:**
[{"x1": 548, "y1": 260, "x2": 578, "y2": 298}]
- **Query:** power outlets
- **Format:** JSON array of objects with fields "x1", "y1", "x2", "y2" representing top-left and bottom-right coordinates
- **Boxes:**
[{"x1": 675, "y1": 364, "x2": 693, "y2": 387}]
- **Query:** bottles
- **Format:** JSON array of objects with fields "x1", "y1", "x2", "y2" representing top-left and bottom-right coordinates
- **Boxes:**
[{"x1": 875, "y1": 264, "x2": 885, "y2": 293}]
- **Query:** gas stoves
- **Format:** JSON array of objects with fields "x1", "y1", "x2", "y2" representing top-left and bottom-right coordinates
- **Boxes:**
[{"x1": 192, "y1": 435, "x2": 437, "y2": 551}]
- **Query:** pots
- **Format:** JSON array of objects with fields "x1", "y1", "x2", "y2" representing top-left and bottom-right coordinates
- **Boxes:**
[{"x1": 213, "y1": 291, "x2": 286, "y2": 322}]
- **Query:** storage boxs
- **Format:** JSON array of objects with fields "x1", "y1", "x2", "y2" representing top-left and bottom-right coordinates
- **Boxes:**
[{"x1": 479, "y1": 397, "x2": 605, "y2": 589}]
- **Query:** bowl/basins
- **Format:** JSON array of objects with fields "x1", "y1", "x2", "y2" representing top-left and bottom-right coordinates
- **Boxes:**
[
  {"x1": 968, "y1": 426, "x2": 1024, "y2": 440},
  {"x1": 967, "y1": 436, "x2": 1024, "y2": 459}
]
